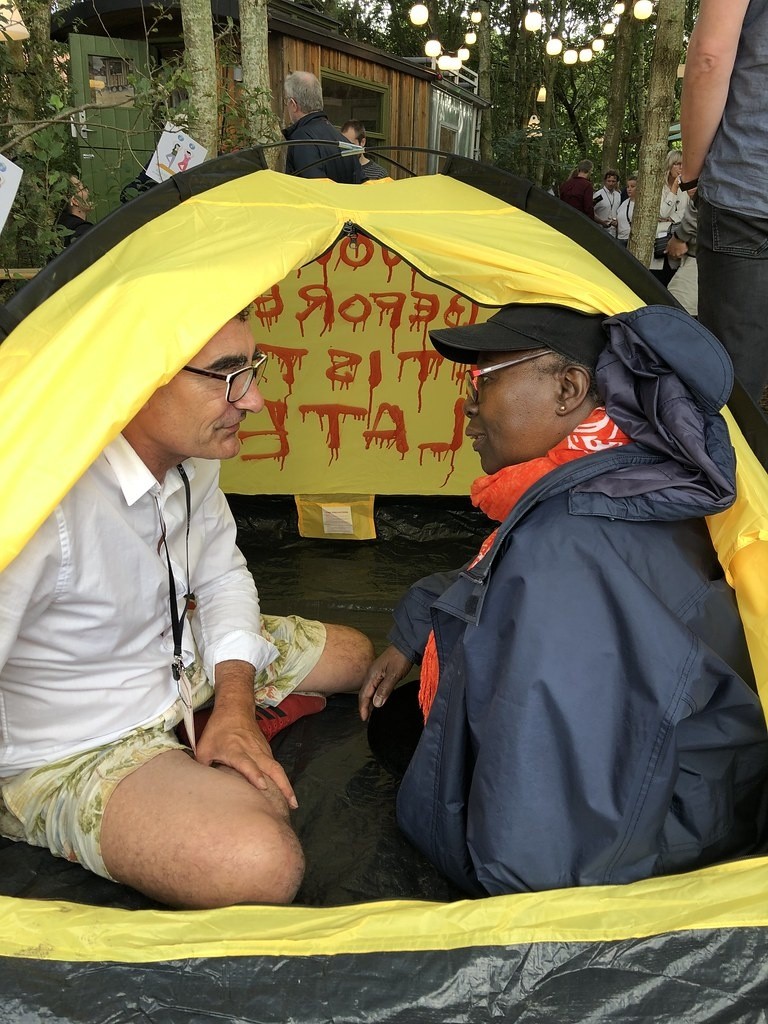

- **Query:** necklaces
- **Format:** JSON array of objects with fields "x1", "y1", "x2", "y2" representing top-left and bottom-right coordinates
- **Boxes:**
[{"x1": 603, "y1": 187, "x2": 615, "y2": 211}]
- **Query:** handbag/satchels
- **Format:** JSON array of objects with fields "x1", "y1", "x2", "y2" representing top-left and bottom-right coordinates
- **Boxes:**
[{"x1": 654, "y1": 236, "x2": 671, "y2": 259}]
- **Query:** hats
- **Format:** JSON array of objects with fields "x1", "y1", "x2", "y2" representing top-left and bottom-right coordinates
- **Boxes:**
[{"x1": 429, "y1": 303, "x2": 608, "y2": 368}]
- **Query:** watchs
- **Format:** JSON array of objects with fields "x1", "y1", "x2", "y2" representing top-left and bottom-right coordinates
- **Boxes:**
[{"x1": 677, "y1": 175, "x2": 698, "y2": 193}]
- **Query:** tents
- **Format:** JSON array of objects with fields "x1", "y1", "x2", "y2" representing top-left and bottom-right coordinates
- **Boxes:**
[{"x1": 1, "y1": 141, "x2": 768, "y2": 1023}]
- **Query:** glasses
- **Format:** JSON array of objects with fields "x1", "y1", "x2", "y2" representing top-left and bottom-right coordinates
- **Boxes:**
[
  {"x1": 183, "y1": 354, "x2": 267, "y2": 404},
  {"x1": 464, "y1": 349, "x2": 554, "y2": 404},
  {"x1": 76, "y1": 184, "x2": 89, "y2": 195}
]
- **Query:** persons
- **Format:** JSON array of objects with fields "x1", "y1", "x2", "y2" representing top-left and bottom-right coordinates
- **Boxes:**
[
  {"x1": 594, "y1": 170, "x2": 621, "y2": 239},
  {"x1": 2, "y1": 303, "x2": 376, "y2": 904},
  {"x1": 566, "y1": 169, "x2": 577, "y2": 182},
  {"x1": 560, "y1": 159, "x2": 594, "y2": 220},
  {"x1": 649, "y1": 148, "x2": 690, "y2": 288},
  {"x1": 679, "y1": 0, "x2": 768, "y2": 413},
  {"x1": 51, "y1": 172, "x2": 97, "y2": 267},
  {"x1": 338, "y1": 120, "x2": 389, "y2": 179},
  {"x1": 655, "y1": 198, "x2": 699, "y2": 321},
  {"x1": 278, "y1": 70, "x2": 365, "y2": 183},
  {"x1": 359, "y1": 305, "x2": 768, "y2": 897},
  {"x1": 617, "y1": 175, "x2": 636, "y2": 246}
]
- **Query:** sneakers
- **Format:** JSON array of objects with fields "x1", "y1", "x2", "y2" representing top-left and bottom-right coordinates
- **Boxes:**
[
  {"x1": 174, "y1": 690, "x2": 327, "y2": 750},
  {"x1": 346, "y1": 754, "x2": 403, "y2": 808}
]
